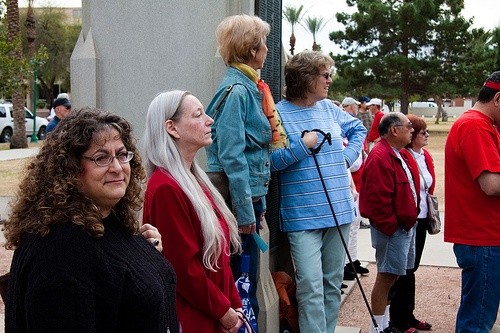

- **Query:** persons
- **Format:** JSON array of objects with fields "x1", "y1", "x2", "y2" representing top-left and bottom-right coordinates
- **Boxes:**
[
  {"x1": 357, "y1": 95, "x2": 373, "y2": 131},
  {"x1": 269, "y1": 51, "x2": 367, "y2": 333},
  {"x1": 359, "y1": 111, "x2": 421, "y2": 333},
  {"x1": 342, "y1": 97, "x2": 361, "y2": 116},
  {"x1": 389, "y1": 113, "x2": 436, "y2": 333},
  {"x1": 444, "y1": 70, "x2": 500, "y2": 333},
  {"x1": 331, "y1": 101, "x2": 369, "y2": 280},
  {"x1": 203, "y1": 13, "x2": 289, "y2": 320},
  {"x1": 46, "y1": 98, "x2": 72, "y2": 134},
  {"x1": 139, "y1": 90, "x2": 244, "y2": 333},
  {"x1": 1, "y1": 106, "x2": 181, "y2": 333},
  {"x1": 367, "y1": 98, "x2": 385, "y2": 155}
]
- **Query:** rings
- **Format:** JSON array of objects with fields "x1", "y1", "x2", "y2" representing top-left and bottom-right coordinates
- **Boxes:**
[{"x1": 153, "y1": 238, "x2": 159, "y2": 246}]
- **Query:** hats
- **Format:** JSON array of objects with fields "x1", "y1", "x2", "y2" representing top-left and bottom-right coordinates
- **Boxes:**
[
  {"x1": 53, "y1": 98, "x2": 71, "y2": 109},
  {"x1": 365, "y1": 98, "x2": 382, "y2": 106},
  {"x1": 359, "y1": 96, "x2": 371, "y2": 102},
  {"x1": 342, "y1": 97, "x2": 361, "y2": 107}
]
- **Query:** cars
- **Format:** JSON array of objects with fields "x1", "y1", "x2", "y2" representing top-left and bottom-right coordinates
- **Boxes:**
[{"x1": 0, "y1": 102, "x2": 50, "y2": 142}]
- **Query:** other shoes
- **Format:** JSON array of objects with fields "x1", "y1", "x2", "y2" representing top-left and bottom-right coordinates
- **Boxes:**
[
  {"x1": 411, "y1": 320, "x2": 432, "y2": 330},
  {"x1": 388, "y1": 321, "x2": 419, "y2": 333},
  {"x1": 360, "y1": 221, "x2": 371, "y2": 228}
]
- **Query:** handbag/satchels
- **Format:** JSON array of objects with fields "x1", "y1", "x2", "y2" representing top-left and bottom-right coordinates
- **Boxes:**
[
  {"x1": 427, "y1": 193, "x2": 441, "y2": 234},
  {"x1": 204, "y1": 170, "x2": 231, "y2": 204}
]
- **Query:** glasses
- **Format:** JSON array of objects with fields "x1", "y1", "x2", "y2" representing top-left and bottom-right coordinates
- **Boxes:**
[
  {"x1": 393, "y1": 123, "x2": 412, "y2": 128},
  {"x1": 417, "y1": 130, "x2": 428, "y2": 135},
  {"x1": 80, "y1": 151, "x2": 134, "y2": 167},
  {"x1": 319, "y1": 72, "x2": 332, "y2": 79}
]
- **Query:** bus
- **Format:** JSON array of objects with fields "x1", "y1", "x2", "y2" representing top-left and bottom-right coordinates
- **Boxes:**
[{"x1": 410, "y1": 97, "x2": 455, "y2": 110}]
